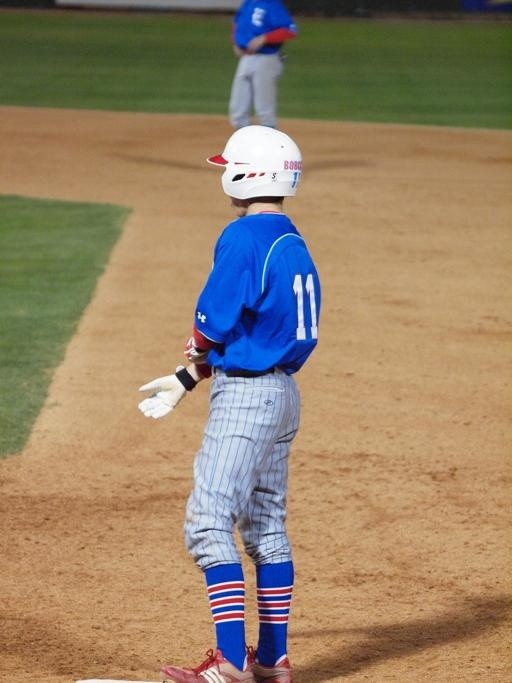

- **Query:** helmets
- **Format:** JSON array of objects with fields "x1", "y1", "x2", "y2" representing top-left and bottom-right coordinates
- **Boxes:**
[{"x1": 205, "y1": 125, "x2": 304, "y2": 202}]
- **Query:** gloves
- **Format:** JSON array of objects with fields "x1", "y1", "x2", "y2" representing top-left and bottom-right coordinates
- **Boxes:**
[{"x1": 136, "y1": 368, "x2": 199, "y2": 422}]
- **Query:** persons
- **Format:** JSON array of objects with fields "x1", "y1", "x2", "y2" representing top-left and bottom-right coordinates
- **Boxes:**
[
  {"x1": 206, "y1": 0, "x2": 299, "y2": 163},
  {"x1": 137, "y1": 123, "x2": 321, "y2": 683}
]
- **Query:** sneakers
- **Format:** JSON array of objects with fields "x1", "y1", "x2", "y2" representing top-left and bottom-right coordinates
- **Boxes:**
[
  {"x1": 246, "y1": 646, "x2": 291, "y2": 683},
  {"x1": 161, "y1": 645, "x2": 255, "y2": 683}
]
